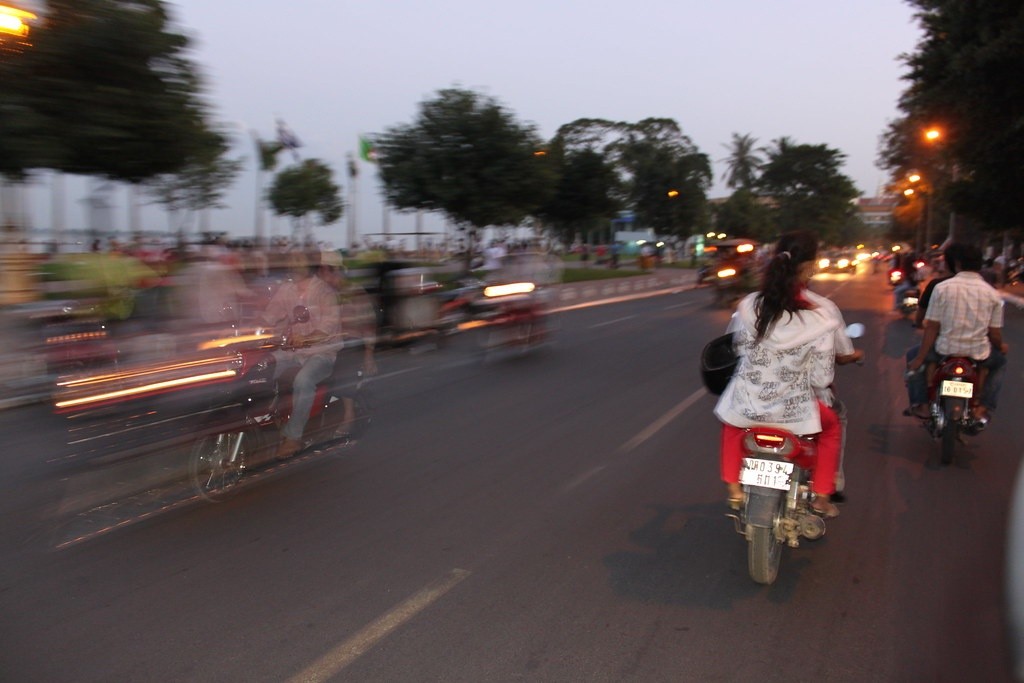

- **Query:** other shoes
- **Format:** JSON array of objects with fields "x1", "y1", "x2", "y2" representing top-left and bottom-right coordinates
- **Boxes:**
[{"x1": 829, "y1": 491, "x2": 849, "y2": 505}]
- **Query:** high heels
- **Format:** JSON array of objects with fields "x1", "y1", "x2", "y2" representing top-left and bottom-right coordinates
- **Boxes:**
[
  {"x1": 813, "y1": 502, "x2": 841, "y2": 520},
  {"x1": 727, "y1": 497, "x2": 745, "y2": 510}
]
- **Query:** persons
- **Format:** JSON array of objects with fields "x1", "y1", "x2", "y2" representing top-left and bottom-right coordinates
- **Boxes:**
[
  {"x1": 34, "y1": 173, "x2": 705, "y2": 494},
  {"x1": 909, "y1": 244, "x2": 1009, "y2": 433},
  {"x1": 803, "y1": 246, "x2": 861, "y2": 504},
  {"x1": 901, "y1": 246, "x2": 984, "y2": 416},
  {"x1": 706, "y1": 231, "x2": 844, "y2": 519},
  {"x1": 794, "y1": 213, "x2": 1024, "y2": 292}
]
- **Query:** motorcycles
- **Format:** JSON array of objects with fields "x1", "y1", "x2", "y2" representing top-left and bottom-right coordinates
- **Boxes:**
[
  {"x1": 724, "y1": 312, "x2": 865, "y2": 585},
  {"x1": 894, "y1": 279, "x2": 919, "y2": 318},
  {"x1": 185, "y1": 305, "x2": 371, "y2": 505},
  {"x1": 910, "y1": 322, "x2": 990, "y2": 462}
]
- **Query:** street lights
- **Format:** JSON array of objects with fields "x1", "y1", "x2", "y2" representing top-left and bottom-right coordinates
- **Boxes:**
[
  {"x1": 904, "y1": 189, "x2": 921, "y2": 256},
  {"x1": 922, "y1": 128, "x2": 959, "y2": 243},
  {"x1": 910, "y1": 173, "x2": 933, "y2": 251}
]
